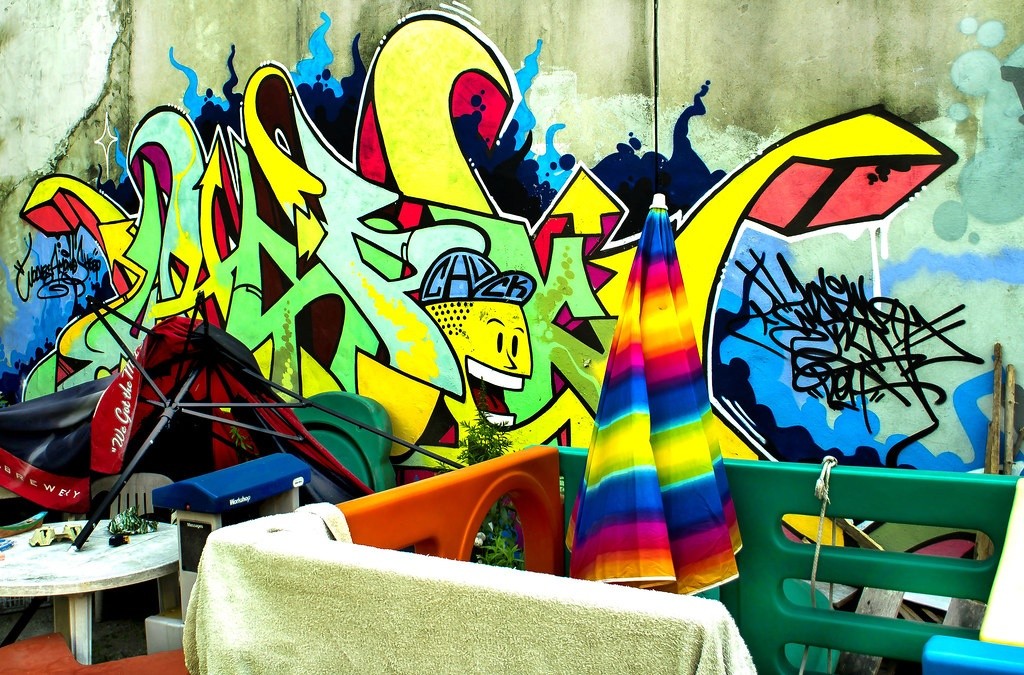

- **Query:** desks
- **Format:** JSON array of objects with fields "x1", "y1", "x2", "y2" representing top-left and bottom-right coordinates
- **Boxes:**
[{"x1": 0, "y1": 520, "x2": 180, "y2": 665}]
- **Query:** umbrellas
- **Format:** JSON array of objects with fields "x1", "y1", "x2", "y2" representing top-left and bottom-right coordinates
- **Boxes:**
[
  {"x1": 0, "y1": 289, "x2": 471, "y2": 652},
  {"x1": 564, "y1": 193, "x2": 745, "y2": 598}
]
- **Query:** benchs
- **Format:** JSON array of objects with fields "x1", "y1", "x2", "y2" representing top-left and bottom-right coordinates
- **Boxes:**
[{"x1": 0, "y1": 632, "x2": 188, "y2": 675}]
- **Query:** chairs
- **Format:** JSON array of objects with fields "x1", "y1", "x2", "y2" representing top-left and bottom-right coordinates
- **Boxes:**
[{"x1": 64, "y1": 472, "x2": 180, "y2": 623}]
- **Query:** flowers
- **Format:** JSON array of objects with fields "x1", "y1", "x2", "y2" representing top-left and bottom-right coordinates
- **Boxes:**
[{"x1": 430, "y1": 376, "x2": 525, "y2": 570}]
